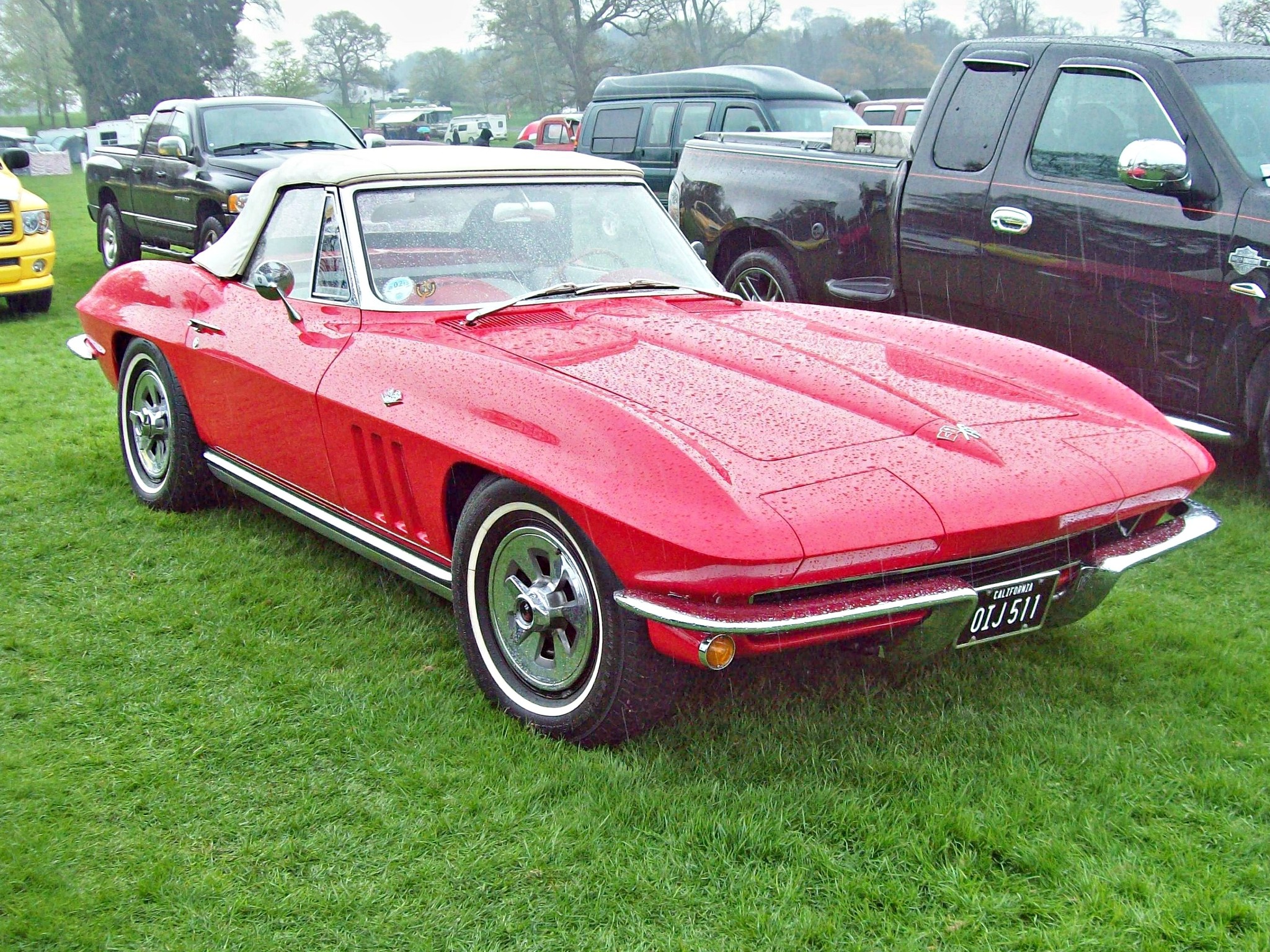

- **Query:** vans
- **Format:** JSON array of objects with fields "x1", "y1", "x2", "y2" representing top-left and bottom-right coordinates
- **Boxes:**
[
  {"x1": 533, "y1": 114, "x2": 582, "y2": 150},
  {"x1": 850, "y1": 98, "x2": 930, "y2": 127},
  {"x1": 443, "y1": 121, "x2": 495, "y2": 145},
  {"x1": 573, "y1": 65, "x2": 877, "y2": 215}
]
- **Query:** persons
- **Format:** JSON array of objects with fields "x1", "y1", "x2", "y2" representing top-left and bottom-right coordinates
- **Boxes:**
[
  {"x1": 473, "y1": 129, "x2": 494, "y2": 147},
  {"x1": 453, "y1": 128, "x2": 460, "y2": 145},
  {"x1": 382, "y1": 124, "x2": 430, "y2": 141}
]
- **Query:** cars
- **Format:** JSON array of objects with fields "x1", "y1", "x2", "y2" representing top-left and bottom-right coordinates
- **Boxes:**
[
  {"x1": 517, "y1": 120, "x2": 540, "y2": 141},
  {"x1": 0, "y1": 148, "x2": 57, "y2": 316},
  {"x1": 66, "y1": 145, "x2": 1226, "y2": 749}
]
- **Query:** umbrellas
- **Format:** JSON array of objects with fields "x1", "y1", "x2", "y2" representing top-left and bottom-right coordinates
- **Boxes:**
[{"x1": 417, "y1": 127, "x2": 431, "y2": 133}]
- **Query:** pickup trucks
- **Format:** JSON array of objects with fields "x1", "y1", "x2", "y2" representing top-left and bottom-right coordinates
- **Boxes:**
[
  {"x1": 84, "y1": 96, "x2": 405, "y2": 272},
  {"x1": 665, "y1": 40, "x2": 1267, "y2": 480}
]
